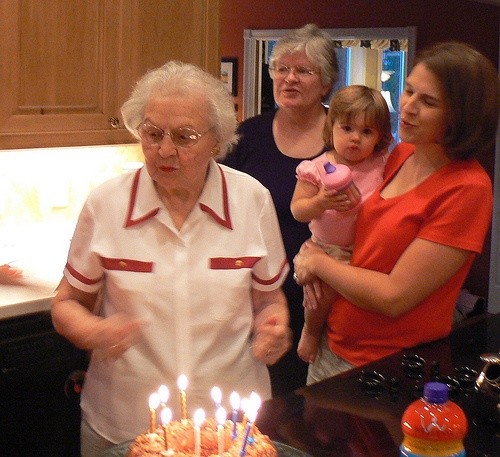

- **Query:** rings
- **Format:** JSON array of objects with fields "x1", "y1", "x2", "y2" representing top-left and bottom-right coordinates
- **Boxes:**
[
  {"x1": 112, "y1": 345, "x2": 120, "y2": 350},
  {"x1": 293, "y1": 273, "x2": 298, "y2": 280},
  {"x1": 267, "y1": 348, "x2": 271, "y2": 356}
]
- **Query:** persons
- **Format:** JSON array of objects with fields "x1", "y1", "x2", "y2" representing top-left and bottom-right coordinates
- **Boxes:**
[
  {"x1": 50, "y1": 60, "x2": 291, "y2": 457},
  {"x1": 0, "y1": 264, "x2": 20, "y2": 284},
  {"x1": 302, "y1": 44, "x2": 494, "y2": 388},
  {"x1": 220, "y1": 24, "x2": 341, "y2": 395},
  {"x1": 291, "y1": 84, "x2": 395, "y2": 364}
]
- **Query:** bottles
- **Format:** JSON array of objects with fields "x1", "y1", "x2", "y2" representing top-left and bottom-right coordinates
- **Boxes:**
[
  {"x1": 399, "y1": 382, "x2": 467, "y2": 457},
  {"x1": 319, "y1": 161, "x2": 361, "y2": 211}
]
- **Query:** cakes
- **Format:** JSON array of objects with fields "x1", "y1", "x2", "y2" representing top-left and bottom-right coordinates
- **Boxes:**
[{"x1": 126, "y1": 416, "x2": 278, "y2": 457}]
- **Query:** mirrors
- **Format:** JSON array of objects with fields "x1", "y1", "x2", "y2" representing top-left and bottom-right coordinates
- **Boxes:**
[{"x1": 243, "y1": 24, "x2": 418, "y2": 153}]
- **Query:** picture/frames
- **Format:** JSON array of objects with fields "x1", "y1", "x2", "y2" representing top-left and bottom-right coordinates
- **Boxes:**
[{"x1": 220, "y1": 57, "x2": 238, "y2": 96}]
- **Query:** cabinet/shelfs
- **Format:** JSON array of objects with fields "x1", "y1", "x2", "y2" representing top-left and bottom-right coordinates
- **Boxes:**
[{"x1": 0, "y1": 0, "x2": 220, "y2": 149}]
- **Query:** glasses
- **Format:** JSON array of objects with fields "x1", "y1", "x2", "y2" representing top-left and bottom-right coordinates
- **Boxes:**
[
  {"x1": 138, "y1": 120, "x2": 215, "y2": 148},
  {"x1": 271, "y1": 63, "x2": 320, "y2": 79}
]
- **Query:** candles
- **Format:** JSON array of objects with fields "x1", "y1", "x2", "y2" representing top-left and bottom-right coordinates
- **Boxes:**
[
  {"x1": 194, "y1": 408, "x2": 205, "y2": 457},
  {"x1": 230, "y1": 390, "x2": 261, "y2": 456},
  {"x1": 158, "y1": 384, "x2": 172, "y2": 453},
  {"x1": 149, "y1": 393, "x2": 160, "y2": 435},
  {"x1": 211, "y1": 386, "x2": 227, "y2": 457},
  {"x1": 177, "y1": 375, "x2": 189, "y2": 424}
]
